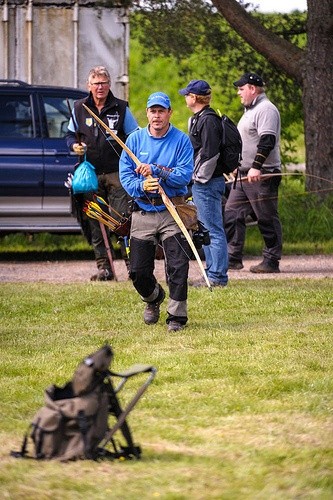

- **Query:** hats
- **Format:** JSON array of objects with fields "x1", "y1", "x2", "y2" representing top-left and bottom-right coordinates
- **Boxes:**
[
  {"x1": 178, "y1": 79, "x2": 211, "y2": 96},
  {"x1": 233, "y1": 72, "x2": 264, "y2": 88},
  {"x1": 146, "y1": 91, "x2": 171, "y2": 109}
]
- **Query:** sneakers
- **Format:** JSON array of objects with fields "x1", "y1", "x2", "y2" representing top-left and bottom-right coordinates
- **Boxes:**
[
  {"x1": 167, "y1": 319, "x2": 185, "y2": 330},
  {"x1": 143, "y1": 285, "x2": 166, "y2": 325}
]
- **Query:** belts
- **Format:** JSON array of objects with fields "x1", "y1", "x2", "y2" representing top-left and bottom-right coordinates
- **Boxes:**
[{"x1": 137, "y1": 197, "x2": 172, "y2": 206}]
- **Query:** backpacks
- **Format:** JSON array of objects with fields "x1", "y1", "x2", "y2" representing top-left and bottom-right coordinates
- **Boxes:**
[
  {"x1": 9, "y1": 336, "x2": 156, "y2": 462},
  {"x1": 195, "y1": 110, "x2": 243, "y2": 174}
]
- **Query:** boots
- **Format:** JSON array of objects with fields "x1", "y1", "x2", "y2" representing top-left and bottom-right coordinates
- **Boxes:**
[
  {"x1": 90, "y1": 257, "x2": 115, "y2": 281},
  {"x1": 124, "y1": 255, "x2": 132, "y2": 280}
]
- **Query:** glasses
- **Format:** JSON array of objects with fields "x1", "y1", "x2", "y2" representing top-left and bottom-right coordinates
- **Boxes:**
[{"x1": 90, "y1": 80, "x2": 110, "y2": 87}]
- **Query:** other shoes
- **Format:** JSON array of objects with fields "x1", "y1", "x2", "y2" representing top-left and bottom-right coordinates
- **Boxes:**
[
  {"x1": 228, "y1": 257, "x2": 243, "y2": 270},
  {"x1": 188, "y1": 273, "x2": 228, "y2": 287},
  {"x1": 249, "y1": 255, "x2": 279, "y2": 273}
]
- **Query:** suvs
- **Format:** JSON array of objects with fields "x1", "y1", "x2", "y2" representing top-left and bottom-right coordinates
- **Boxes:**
[{"x1": 0, "y1": 77, "x2": 236, "y2": 260}]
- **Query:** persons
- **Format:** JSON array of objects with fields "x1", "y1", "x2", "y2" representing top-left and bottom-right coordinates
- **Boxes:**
[
  {"x1": 179, "y1": 80, "x2": 230, "y2": 285},
  {"x1": 221, "y1": 74, "x2": 283, "y2": 273},
  {"x1": 118, "y1": 92, "x2": 195, "y2": 332},
  {"x1": 64, "y1": 65, "x2": 140, "y2": 278}
]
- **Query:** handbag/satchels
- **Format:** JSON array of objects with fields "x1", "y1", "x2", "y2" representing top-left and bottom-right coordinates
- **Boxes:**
[{"x1": 72, "y1": 148, "x2": 98, "y2": 195}]
